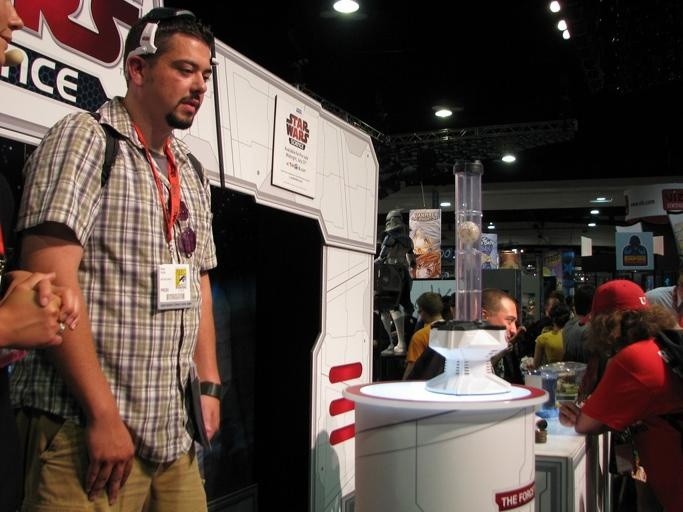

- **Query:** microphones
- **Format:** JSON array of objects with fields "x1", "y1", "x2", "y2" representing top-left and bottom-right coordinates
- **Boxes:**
[{"x1": 3, "y1": 49, "x2": 24, "y2": 67}]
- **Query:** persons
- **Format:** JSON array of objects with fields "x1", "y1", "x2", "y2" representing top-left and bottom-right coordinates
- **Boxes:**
[
  {"x1": 0, "y1": 1, "x2": 81, "y2": 370},
  {"x1": 376, "y1": 206, "x2": 416, "y2": 353},
  {"x1": 13, "y1": 8, "x2": 221, "y2": 511}
]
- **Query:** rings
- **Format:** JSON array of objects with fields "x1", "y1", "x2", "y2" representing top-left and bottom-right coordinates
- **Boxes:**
[{"x1": 59, "y1": 321, "x2": 66, "y2": 334}]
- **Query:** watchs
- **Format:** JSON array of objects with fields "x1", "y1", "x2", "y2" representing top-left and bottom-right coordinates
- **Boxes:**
[{"x1": 200, "y1": 380, "x2": 226, "y2": 400}]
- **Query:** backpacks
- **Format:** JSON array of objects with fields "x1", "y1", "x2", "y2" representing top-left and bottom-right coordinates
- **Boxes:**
[{"x1": 654, "y1": 325, "x2": 683, "y2": 435}]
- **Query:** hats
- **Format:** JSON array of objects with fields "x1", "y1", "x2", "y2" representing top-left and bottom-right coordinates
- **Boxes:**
[{"x1": 578, "y1": 279, "x2": 650, "y2": 325}]
